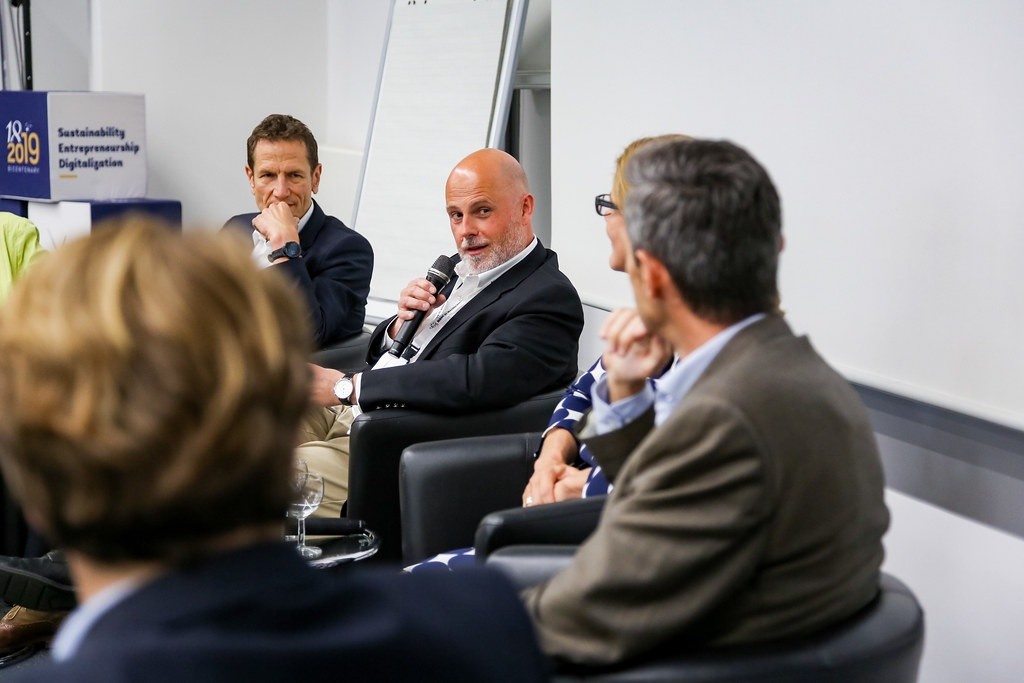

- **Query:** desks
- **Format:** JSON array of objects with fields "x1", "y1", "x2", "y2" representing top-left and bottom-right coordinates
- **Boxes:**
[{"x1": 299, "y1": 514, "x2": 384, "y2": 569}]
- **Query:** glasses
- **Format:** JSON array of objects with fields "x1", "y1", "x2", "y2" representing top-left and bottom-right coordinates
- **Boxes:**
[{"x1": 595, "y1": 194, "x2": 620, "y2": 216}]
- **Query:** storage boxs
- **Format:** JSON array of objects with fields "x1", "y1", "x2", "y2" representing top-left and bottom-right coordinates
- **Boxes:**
[{"x1": 0, "y1": 90, "x2": 185, "y2": 258}]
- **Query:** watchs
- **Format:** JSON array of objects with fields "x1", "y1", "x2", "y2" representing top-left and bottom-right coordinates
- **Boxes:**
[
  {"x1": 268, "y1": 241, "x2": 301, "y2": 262},
  {"x1": 334, "y1": 372, "x2": 356, "y2": 406}
]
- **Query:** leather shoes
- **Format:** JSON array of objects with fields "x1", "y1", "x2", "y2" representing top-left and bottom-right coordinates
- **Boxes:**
[
  {"x1": 0, "y1": 550, "x2": 75, "y2": 612},
  {"x1": 0, "y1": 604, "x2": 68, "y2": 669}
]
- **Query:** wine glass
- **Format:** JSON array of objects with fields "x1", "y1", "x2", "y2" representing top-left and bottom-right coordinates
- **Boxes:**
[{"x1": 289, "y1": 473, "x2": 325, "y2": 558}]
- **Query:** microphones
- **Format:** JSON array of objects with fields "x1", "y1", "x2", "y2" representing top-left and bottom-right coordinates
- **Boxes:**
[
  {"x1": 284, "y1": 511, "x2": 364, "y2": 536},
  {"x1": 389, "y1": 255, "x2": 456, "y2": 359}
]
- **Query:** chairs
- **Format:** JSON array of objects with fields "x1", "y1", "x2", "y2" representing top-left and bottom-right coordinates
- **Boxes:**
[{"x1": 304, "y1": 311, "x2": 930, "y2": 683}]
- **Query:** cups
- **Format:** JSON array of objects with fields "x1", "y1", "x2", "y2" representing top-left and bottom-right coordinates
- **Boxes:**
[{"x1": 296, "y1": 460, "x2": 309, "y2": 490}]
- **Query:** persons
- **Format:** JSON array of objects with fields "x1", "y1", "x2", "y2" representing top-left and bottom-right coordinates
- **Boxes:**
[
  {"x1": 0, "y1": 149, "x2": 585, "y2": 613},
  {"x1": 0, "y1": 212, "x2": 46, "y2": 308},
  {"x1": 0, "y1": 214, "x2": 549, "y2": 683},
  {"x1": 522, "y1": 139, "x2": 889, "y2": 674},
  {"x1": 404, "y1": 134, "x2": 695, "y2": 570},
  {"x1": 0, "y1": 115, "x2": 374, "y2": 667}
]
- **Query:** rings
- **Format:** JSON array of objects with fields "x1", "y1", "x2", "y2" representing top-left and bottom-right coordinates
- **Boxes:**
[{"x1": 526, "y1": 497, "x2": 533, "y2": 503}]
